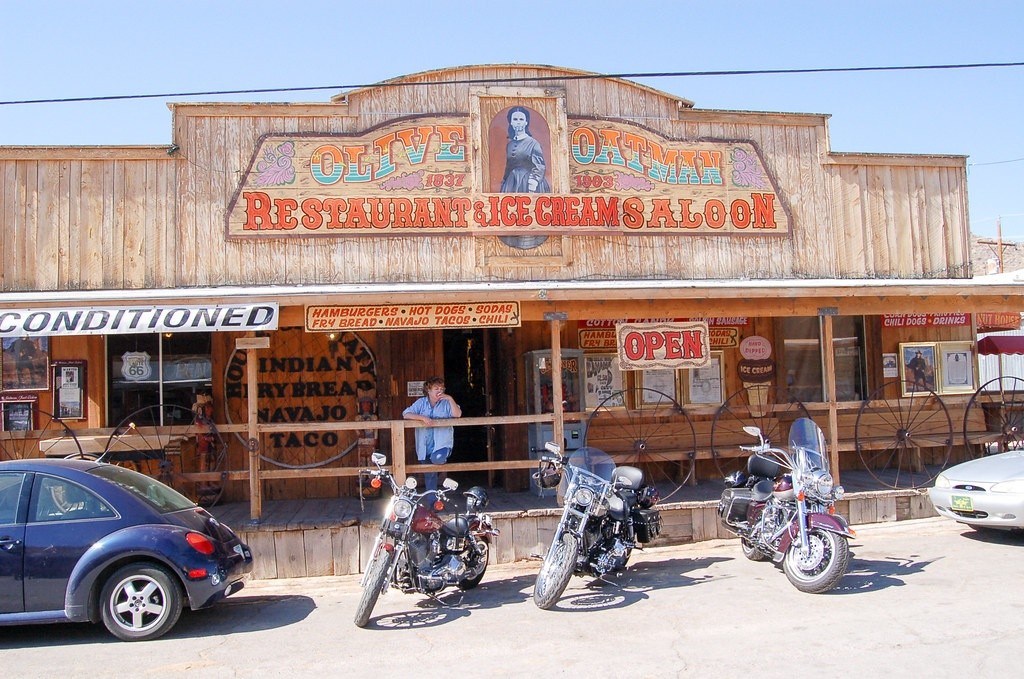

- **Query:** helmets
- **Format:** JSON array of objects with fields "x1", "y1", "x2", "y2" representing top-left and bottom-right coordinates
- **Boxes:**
[
  {"x1": 463, "y1": 486, "x2": 488, "y2": 507},
  {"x1": 532, "y1": 466, "x2": 562, "y2": 488},
  {"x1": 772, "y1": 474, "x2": 794, "y2": 492}
]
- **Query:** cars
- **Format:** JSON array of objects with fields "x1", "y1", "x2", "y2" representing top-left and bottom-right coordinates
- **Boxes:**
[
  {"x1": 927, "y1": 446, "x2": 1024, "y2": 533},
  {"x1": 0, "y1": 455, "x2": 254, "y2": 643}
]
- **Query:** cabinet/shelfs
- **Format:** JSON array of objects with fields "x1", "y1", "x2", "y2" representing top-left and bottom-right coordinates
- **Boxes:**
[{"x1": 523, "y1": 347, "x2": 590, "y2": 498}]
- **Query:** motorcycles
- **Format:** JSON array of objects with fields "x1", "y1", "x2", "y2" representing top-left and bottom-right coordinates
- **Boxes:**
[
  {"x1": 529, "y1": 441, "x2": 666, "y2": 612},
  {"x1": 714, "y1": 416, "x2": 858, "y2": 595},
  {"x1": 352, "y1": 449, "x2": 502, "y2": 627}
]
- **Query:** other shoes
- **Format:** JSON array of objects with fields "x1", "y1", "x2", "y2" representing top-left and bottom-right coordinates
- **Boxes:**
[{"x1": 429, "y1": 504, "x2": 441, "y2": 513}]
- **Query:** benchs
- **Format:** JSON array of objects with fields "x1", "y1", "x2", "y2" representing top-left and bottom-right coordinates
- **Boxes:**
[{"x1": 588, "y1": 407, "x2": 1024, "y2": 489}]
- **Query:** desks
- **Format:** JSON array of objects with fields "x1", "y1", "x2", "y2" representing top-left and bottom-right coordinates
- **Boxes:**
[{"x1": 39, "y1": 434, "x2": 188, "y2": 473}]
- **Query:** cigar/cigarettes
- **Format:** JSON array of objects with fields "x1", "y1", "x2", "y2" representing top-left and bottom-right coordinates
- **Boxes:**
[{"x1": 437, "y1": 391, "x2": 441, "y2": 396}]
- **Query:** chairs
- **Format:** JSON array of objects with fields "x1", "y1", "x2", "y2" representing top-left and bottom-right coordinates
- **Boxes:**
[
  {"x1": 61, "y1": 486, "x2": 91, "y2": 520},
  {"x1": 36, "y1": 485, "x2": 51, "y2": 522}
]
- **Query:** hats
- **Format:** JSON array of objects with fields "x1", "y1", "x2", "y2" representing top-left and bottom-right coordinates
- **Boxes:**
[{"x1": 915, "y1": 350, "x2": 922, "y2": 355}]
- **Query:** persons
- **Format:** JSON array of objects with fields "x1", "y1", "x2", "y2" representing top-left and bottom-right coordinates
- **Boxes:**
[
  {"x1": 908, "y1": 351, "x2": 931, "y2": 391},
  {"x1": 192, "y1": 394, "x2": 223, "y2": 507},
  {"x1": 402, "y1": 376, "x2": 462, "y2": 512},
  {"x1": 11, "y1": 338, "x2": 37, "y2": 385},
  {"x1": 495, "y1": 107, "x2": 551, "y2": 248},
  {"x1": 355, "y1": 387, "x2": 379, "y2": 500}
]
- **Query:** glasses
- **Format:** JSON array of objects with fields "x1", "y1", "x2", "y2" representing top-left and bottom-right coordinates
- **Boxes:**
[{"x1": 434, "y1": 386, "x2": 447, "y2": 392}]
